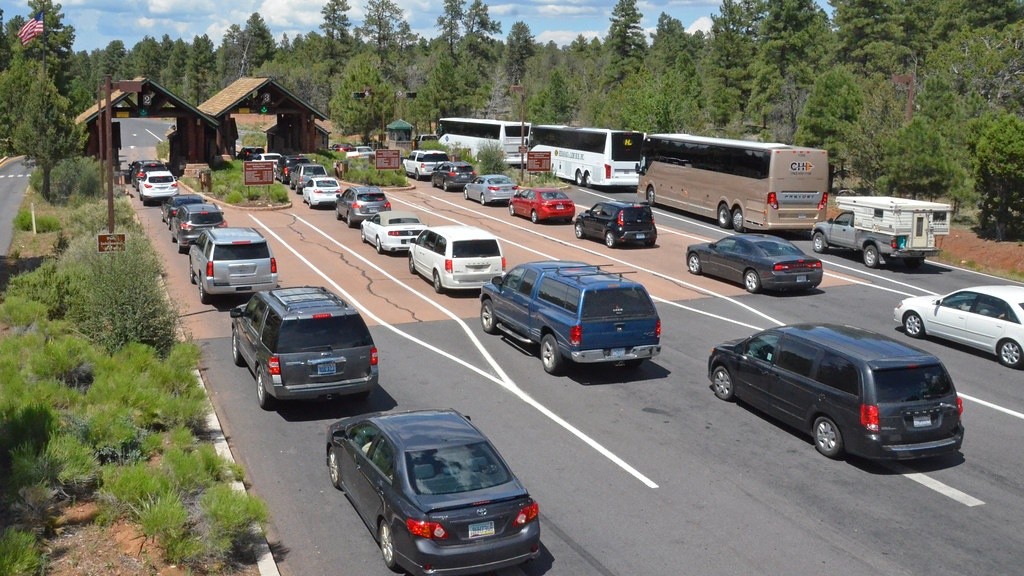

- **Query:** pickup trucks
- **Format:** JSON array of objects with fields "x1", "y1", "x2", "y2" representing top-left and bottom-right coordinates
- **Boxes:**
[{"x1": 811, "y1": 211, "x2": 943, "y2": 267}]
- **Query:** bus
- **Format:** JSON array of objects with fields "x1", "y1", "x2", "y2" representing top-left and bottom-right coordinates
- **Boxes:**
[
  {"x1": 437, "y1": 118, "x2": 531, "y2": 165},
  {"x1": 525, "y1": 124, "x2": 648, "y2": 189},
  {"x1": 634, "y1": 132, "x2": 829, "y2": 232}
]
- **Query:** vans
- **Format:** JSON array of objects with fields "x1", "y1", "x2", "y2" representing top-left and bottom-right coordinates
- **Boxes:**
[
  {"x1": 408, "y1": 226, "x2": 507, "y2": 294},
  {"x1": 706, "y1": 321, "x2": 965, "y2": 463},
  {"x1": 480, "y1": 259, "x2": 662, "y2": 374}
]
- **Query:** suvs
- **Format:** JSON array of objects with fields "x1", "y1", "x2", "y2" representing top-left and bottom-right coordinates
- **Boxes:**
[
  {"x1": 186, "y1": 224, "x2": 278, "y2": 303},
  {"x1": 230, "y1": 286, "x2": 379, "y2": 410},
  {"x1": 337, "y1": 185, "x2": 390, "y2": 227},
  {"x1": 401, "y1": 150, "x2": 449, "y2": 181}
]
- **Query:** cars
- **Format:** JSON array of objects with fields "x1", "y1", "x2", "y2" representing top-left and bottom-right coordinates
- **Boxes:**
[
  {"x1": 686, "y1": 234, "x2": 824, "y2": 293},
  {"x1": 237, "y1": 146, "x2": 341, "y2": 209},
  {"x1": 326, "y1": 406, "x2": 542, "y2": 576},
  {"x1": 431, "y1": 162, "x2": 659, "y2": 248},
  {"x1": 895, "y1": 285, "x2": 1024, "y2": 368},
  {"x1": 128, "y1": 158, "x2": 226, "y2": 253},
  {"x1": 362, "y1": 211, "x2": 427, "y2": 256},
  {"x1": 332, "y1": 134, "x2": 436, "y2": 159}
]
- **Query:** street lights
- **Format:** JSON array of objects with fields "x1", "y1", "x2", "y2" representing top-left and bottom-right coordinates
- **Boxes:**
[{"x1": 105, "y1": 81, "x2": 143, "y2": 234}]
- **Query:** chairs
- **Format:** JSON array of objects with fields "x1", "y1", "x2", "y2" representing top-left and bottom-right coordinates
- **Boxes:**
[
  {"x1": 501, "y1": 179, "x2": 508, "y2": 184},
  {"x1": 556, "y1": 193, "x2": 563, "y2": 199},
  {"x1": 414, "y1": 464, "x2": 437, "y2": 479},
  {"x1": 488, "y1": 179, "x2": 495, "y2": 184},
  {"x1": 461, "y1": 457, "x2": 489, "y2": 473},
  {"x1": 542, "y1": 194, "x2": 549, "y2": 199}
]
